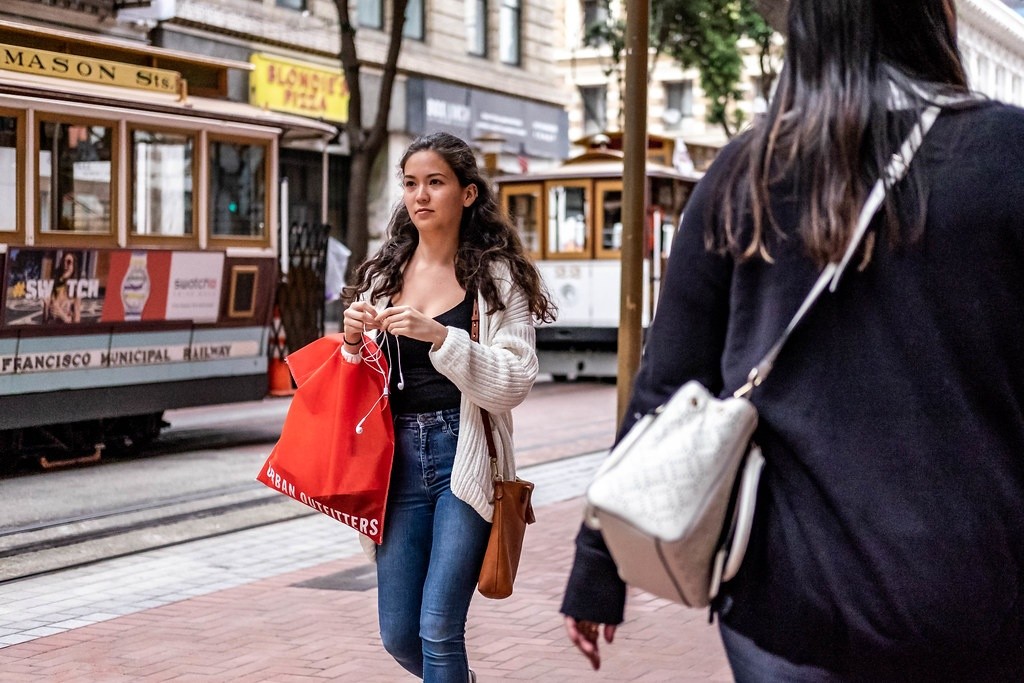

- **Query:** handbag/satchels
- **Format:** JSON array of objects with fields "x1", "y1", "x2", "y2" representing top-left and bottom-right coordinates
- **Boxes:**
[
  {"x1": 258, "y1": 333, "x2": 394, "y2": 546},
  {"x1": 583, "y1": 378, "x2": 766, "y2": 609},
  {"x1": 477, "y1": 477, "x2": 537, "y2": 599}
]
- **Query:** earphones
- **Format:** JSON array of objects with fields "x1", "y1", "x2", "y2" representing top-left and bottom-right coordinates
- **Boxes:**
[
  {"x1": 397, "y1": 373, "x2": 405, "y2": 390},
  {"x1": 356, "y1": 417, "x2": 366, "y2": 434}
]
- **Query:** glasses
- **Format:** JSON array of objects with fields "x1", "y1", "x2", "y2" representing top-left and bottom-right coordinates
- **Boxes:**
[{"x1": 62, "y1": 260, "x2": 74, "y2": 265}]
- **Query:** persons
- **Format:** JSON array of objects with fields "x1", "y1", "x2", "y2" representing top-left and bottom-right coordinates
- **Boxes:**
[
  {"x1": 43, "y1": 252, "x2": 80, "y2": 325},
  {"x1": 341, "y1": 132, "x2": 558, "y2": 683},
  {"x1": 559, "y1": 0, "x2": 1024, "y2": 683}
]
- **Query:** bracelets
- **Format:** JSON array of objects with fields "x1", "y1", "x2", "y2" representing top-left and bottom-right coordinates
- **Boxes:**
[{"x1": 343, "y1": 337, "x2": 362, "y2": 345}]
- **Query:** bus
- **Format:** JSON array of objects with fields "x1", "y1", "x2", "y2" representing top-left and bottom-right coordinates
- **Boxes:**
[
  {"x1": 496, "y1": 132, "x2": 723, "y2": 382},
  {"x1": 0, "y1": 19, "x2": 337, "y2": 474}
]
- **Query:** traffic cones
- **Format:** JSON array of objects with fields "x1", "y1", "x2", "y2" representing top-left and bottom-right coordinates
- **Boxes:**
[{"x1": 267, "y1": 306, "x2": 298, "y2": 398}]
